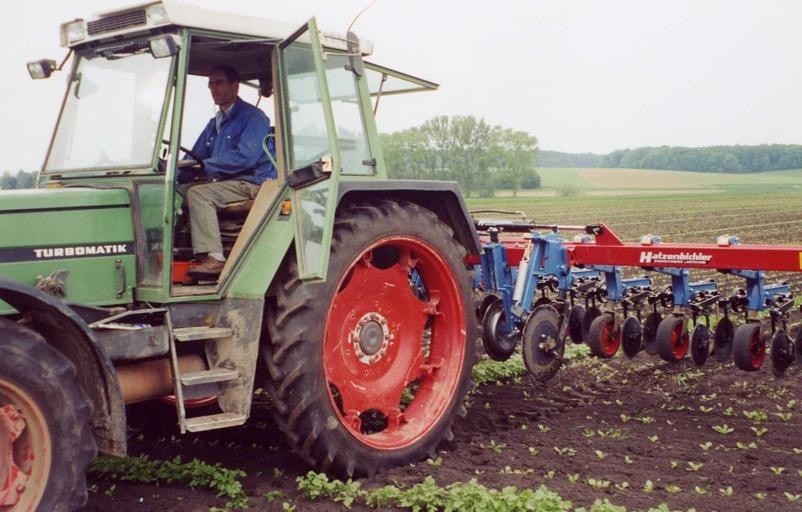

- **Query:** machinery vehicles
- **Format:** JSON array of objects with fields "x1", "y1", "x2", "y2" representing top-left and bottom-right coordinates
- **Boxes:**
[{"x1": 2, "y1": 0, "x2": 484, "y2": 511}]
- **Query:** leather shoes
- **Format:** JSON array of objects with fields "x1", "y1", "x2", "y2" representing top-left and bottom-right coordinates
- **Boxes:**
[{"x1": 187, "y1": 256, "x2": 226, "y2": 277}]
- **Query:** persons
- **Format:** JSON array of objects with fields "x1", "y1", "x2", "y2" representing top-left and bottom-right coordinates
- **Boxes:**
[{"x1": 161, "y1": 65, "x2": 277, "y2": 277}]
